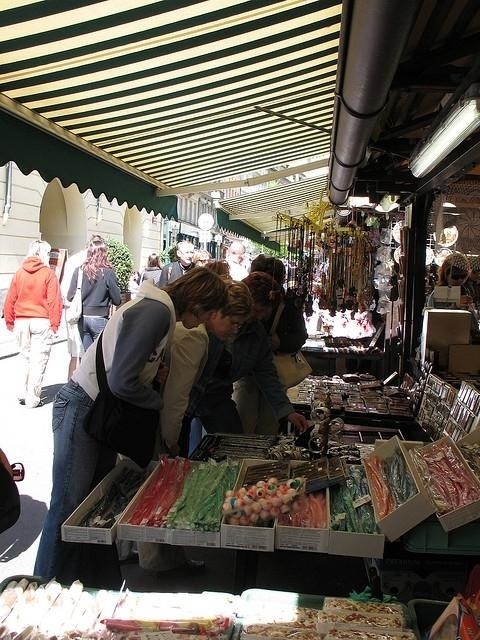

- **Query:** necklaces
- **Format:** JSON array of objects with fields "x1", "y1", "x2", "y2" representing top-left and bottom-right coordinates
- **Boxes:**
[{"x1": 269, "y1": 212, "x2": 376, "y2": 318}]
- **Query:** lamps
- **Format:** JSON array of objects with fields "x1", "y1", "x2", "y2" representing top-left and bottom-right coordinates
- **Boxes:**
[{"x1": 408, "y1": 96, "x2": 480, "y2": 180}]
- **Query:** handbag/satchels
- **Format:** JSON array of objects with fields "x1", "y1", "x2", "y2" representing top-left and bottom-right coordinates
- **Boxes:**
[
  {"x1": 82, "y1": 388, "x2": 160, "y2": 469},
  {"x1": 63, "y1": 265, "x2": 84, "y2": 326},
  {"x1": 271, "y1": 349, "x2": 313, "y2": 392}
]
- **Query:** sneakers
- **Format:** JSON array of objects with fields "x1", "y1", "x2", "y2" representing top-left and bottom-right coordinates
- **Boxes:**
[{"x1": 17, "y1": 395, "x2": 45, "y2": 408}]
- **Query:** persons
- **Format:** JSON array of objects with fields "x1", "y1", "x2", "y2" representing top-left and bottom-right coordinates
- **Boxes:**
[
  {"x1": 3, "y1": 234, "x2": 212, "y2": 409},
  {"x1": 32, "y1": 253, "x2": 309, "y2": 589},
  {"x1": 225, "y1": 241, "x2": 249, "y2": 284},
  {"x1": 426, "y1": 251, "x2": 480, "y2": 310}
]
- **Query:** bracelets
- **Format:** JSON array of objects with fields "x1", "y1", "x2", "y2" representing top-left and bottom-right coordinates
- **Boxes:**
[{"x1": 310, "y1": 399, "x2": 343, "y2": 454}]
